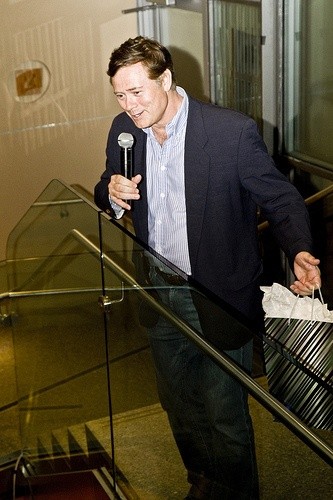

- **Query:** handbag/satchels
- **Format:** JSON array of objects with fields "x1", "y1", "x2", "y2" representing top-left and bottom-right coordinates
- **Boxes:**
[{"x1": 263, "y1": 284, "x2": 333, "y2": 433}]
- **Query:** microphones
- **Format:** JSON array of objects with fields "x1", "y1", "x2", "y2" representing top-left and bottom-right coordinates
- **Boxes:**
[{"x1": 117, "y1": 132, "x2": 135, "y2": 212}]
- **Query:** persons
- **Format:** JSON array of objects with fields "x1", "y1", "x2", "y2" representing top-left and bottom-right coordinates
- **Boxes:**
[{"x1": 93, "y1": 36, "x2": 321, "y2": 500}]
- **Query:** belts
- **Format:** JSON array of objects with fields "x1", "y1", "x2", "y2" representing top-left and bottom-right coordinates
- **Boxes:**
[{"x1": 154, "y1": 266, "x2": 190, "y2": 287}]
